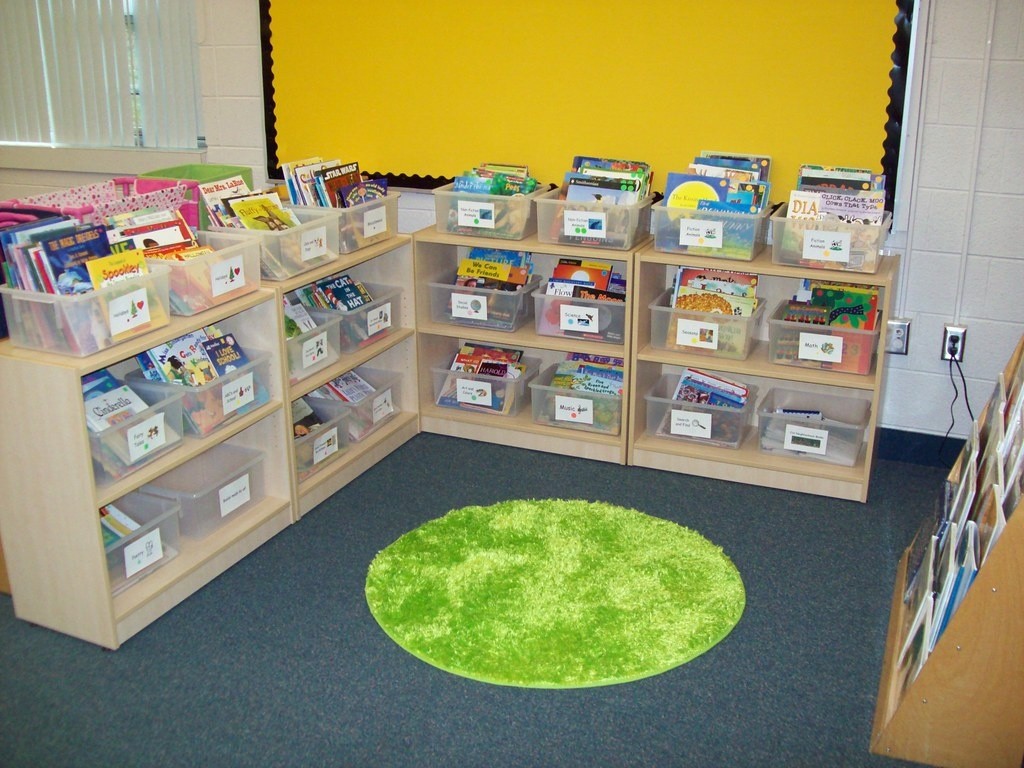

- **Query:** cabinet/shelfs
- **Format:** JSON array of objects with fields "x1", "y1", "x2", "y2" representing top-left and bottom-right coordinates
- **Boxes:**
[
  {"x1": 414, "y1": 223, "x2": 654, "y2": 467},
  {"x1": 255, "y1": 232, "x2": 420, "y2": 524},
  {"x1": 626, "y1": 236, "x2": 901, "y2": 505},
  {"x1": 867, "y1": 330, "x2": 1024, "y2": 768},
  {"x1": 0, "y1": 286, "x2": 296, "y2": 653}
]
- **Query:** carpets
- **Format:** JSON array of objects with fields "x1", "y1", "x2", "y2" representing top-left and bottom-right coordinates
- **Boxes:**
[{"x1": 362, "y1": 497, "x2": 747, "y2": 692}]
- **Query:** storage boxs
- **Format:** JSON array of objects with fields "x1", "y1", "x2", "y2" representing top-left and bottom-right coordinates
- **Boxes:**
[
  {"x1": 0, "y1": 263, "x2": 173, "y2": 359},
  {"x1": 88, "y1": 378, "x2": 186, "y2": 490},
  {"x1": 282, "y1": 190, "x2": 401, "y2": 255},
  {"x1": 208, "y1": 208, "x2": 343, "y2": 282},
  {"x1": 0, "y1": 175, "x2": 200, "y2": 229},
  {"x1": 531, "y1": 284, "x2": 626, "y2": 346},
  {"x1": 530, "y1": 188, "x2": 656, "y2": 252},
  {"x1": 285, "y1": 309, "x2": 345, "y2": 388},
  {"x1": 647, "y1": 288, "x2": 768, "y2": 361},
  {"x1": 650, "y1": 198, "x2": 774, "y2": 262},
  {"x1": 136, "y1": 440, "x2": 267, "y2": 544},
  {"x1": 430, "y1": 178, "x2": 553, "y2": 242},
  {"x1": 429, "y1": 344, "x2": 543, "y2": 418},
  {"x1": 425, "y1": 266, "x2": 541, "y2": 334},
  {"x1": 145, "y1": 230, "x2": 261, "y2": 317},
  {"x1": 122, "y1": 346, "x2": 273, "y2": 439},
  {"x1": 138, "y1": 162, "x2": 254, "y2": 232},
  {"x1": 756, "y1": 386, "x2": 871, "y2": 465},
  {"x1": 302, "y1": 365, "x2": 404, "y2": 443},
  {"x1": 642, "y1": 373, "x2": 760, "y2": 450},
  {"x1": 294, "y1": 399, "x2": 352, "y2": 484},
  {"x1": 769, "y1": 202, "x2": 893, "y2": 275},
  {"x1": 290, "y1": 281, "x2": 404, "y2": 355},
  {"x1": 526, "y1": 361, "x2": 622, "y2": 436},
  {"x1": 105, "y1": 491, "x2": 182, "y2": 598},
  {"x1": 764, "y1": 298, "x2": 882, "y2": 375}
]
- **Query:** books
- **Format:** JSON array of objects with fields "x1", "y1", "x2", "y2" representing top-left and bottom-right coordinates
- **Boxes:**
[
  {"x1": 0, "y1": 158, "x2": 400, "y2": 583},
  {"x1": 435, "y1": 152, "x2": 886, "y2": 467},
  {"x1": 896, "y1": 360, "x2": 1024, "y2": 691}
]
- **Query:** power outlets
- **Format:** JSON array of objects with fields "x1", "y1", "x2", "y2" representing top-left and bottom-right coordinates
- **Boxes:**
[
  {"x1": 885, "y1": 320, "x2": 911, "y2": 356},
  {"x1": 940, "y1": 326, "x2": 967, "y2": 363}
]
